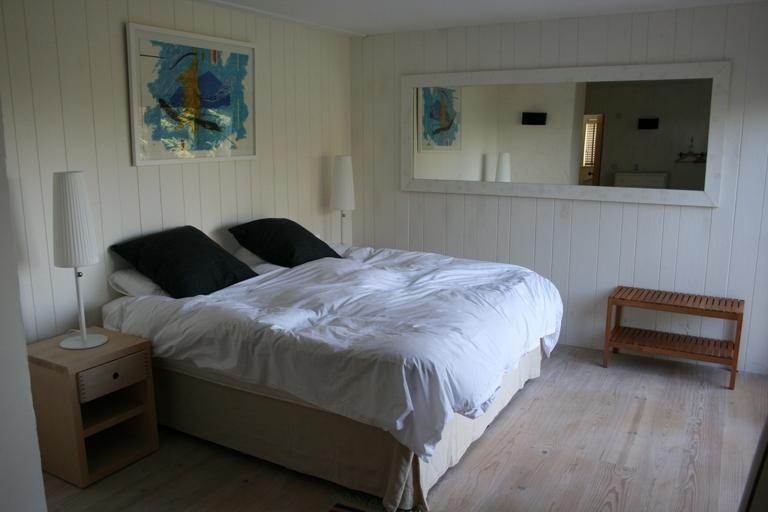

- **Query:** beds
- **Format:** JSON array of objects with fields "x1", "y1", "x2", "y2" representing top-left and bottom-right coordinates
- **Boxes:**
[{"x1": 101, "y1": 241, "x2": 564, "y2": 512}]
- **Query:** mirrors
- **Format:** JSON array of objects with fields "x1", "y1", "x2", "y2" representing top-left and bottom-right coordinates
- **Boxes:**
[{"x1": 400, "y1": 59, "x2": 732, "y2": 208}]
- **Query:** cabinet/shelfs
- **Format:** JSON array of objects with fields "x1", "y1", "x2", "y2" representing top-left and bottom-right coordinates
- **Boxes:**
[
  {"x1": 615, "y1": 172, "x2": 671, "y2": 189},
  {"x1": 603, "y1": 286, "x2": 745, "y2": 390}
]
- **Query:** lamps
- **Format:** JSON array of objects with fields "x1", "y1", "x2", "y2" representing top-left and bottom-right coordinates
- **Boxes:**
[
  {"x1": 495, "y1": 152, "x2": 512, "y2": 183},
  {"x1": 53, "y1": 170, "x2": 108, "y2": 351},
  {"x1": 330, "y1": 155, "x2": 355, "y2": 245}
]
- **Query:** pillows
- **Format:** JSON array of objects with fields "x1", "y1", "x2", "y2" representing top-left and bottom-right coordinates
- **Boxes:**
[{"x1": 108, "y1": 218, "x2": 342, "y2": 299}]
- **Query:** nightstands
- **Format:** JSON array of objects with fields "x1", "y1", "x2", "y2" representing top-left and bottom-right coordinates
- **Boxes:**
[{"x1": 26, "y1": 325, "x2": 160, "y2": 489}]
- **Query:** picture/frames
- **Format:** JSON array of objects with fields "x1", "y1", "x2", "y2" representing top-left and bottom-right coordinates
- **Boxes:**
[
  {"x1": 125, "y1": 22, "x2": 260, "y2": 166},
  {"x1": 417, "y1": 87, "x2": 462, "y2": 154}
]
